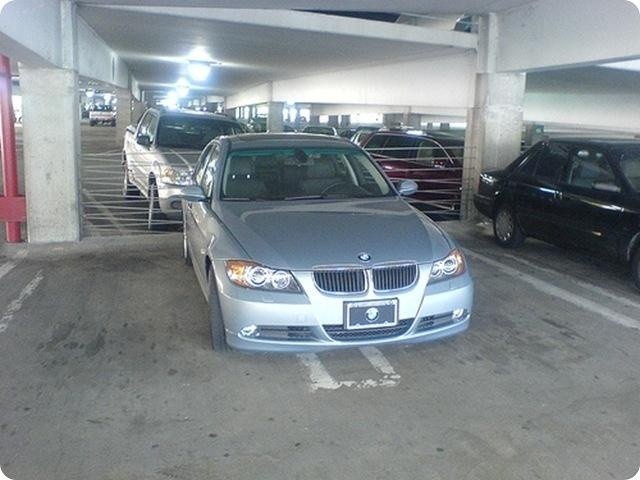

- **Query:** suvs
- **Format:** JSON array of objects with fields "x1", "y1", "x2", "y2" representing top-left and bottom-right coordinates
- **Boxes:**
[{"x1": 354, "y1": 127, "x2": 467, "y2": 223}]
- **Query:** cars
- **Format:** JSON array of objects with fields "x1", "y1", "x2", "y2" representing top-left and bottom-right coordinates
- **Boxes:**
[
  {"x1": 248, "y1": 111, "x2": 465, "y2": 166},
  {"x1": 472, "y1": 135, "x2": 640, "y2": 292},
  {"x1": 177, "y1": 129, "x2": 478, "y2": 359}
]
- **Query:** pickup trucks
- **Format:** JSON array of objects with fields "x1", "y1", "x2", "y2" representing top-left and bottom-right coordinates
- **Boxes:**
[
  {"x1": 121, "y1": 104, "x2": 258, "y2": 232},
  {"x1": 88, "y1": 103, "x2": 117, "y2": 128}
]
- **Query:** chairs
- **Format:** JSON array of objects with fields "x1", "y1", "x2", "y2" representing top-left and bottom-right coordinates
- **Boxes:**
[
  {"x1": 298, "y1": 159, "x2": 345, "y2": 195},
  {"x1": 226, "y1": 158, "x2": 267, "y2": 198},
  {"x1": 575, "y1": 161, "x2": 608, "y2": 189},
  {"x1": 624, "y1": 159, "x2": 640, "y2": 189}
]
